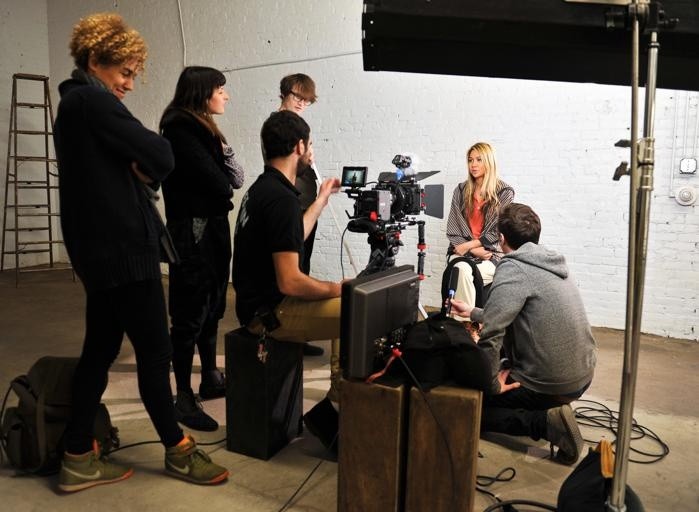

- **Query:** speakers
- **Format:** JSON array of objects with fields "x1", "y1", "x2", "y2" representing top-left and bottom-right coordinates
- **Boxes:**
[{"x1": 223, "y1": 327, "x2": 303, "y2": 461}]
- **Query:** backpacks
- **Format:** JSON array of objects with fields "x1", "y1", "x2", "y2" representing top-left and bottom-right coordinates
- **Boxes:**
[{"x1": 0, "y1": 354, "x2": 120, "y2": 477}]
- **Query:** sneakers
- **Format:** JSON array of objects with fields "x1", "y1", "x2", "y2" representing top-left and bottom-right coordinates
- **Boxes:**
[
  {"x1": 55, "y1": 449, "x2": 135, "y2": 494},
  {"x1": 163, "y1": 434, "x2": 229, "y2": 486},
  {"x1": 175, "y1": 391, "x2": 218, "y2": 432},
  {"x1": 545, "y1": 404, "x2": 582, "y2": 467},
  {"x1": 199, "y1": 367, "x2": 226, "y2": 401},
  {"x1": 301, "y1": 396, "x2": 339, "y2": 461}
]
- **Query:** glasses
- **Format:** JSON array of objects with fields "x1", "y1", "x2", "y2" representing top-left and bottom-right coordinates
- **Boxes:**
[{"x1": 289, "y1": 91, "x2": 313, "y2": 107}]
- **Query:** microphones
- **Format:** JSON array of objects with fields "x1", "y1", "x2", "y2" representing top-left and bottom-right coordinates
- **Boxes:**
[{"x1": 446, "y1": 267, "x2": 459, "y2": 318}]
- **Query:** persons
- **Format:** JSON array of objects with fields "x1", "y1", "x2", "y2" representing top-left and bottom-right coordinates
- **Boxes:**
[
  {"x1": 231, "y1": 109, "x2": 354, "y2": 460},
  {"x1": 443, "y1": 201, "x2": 597, "y2": 466},
  {"x1": 259, "y1": 73, "x2": 326, "y2": 357},
  {"x1": 438, "y1": 142, "x2": 517, "y2": 344},
  {"x1": 49, "y1": 9, "x2": 231, "y2": 495},
  {"x1": 157, "y1": 65, "x2": 244, "y2": 433}
]
